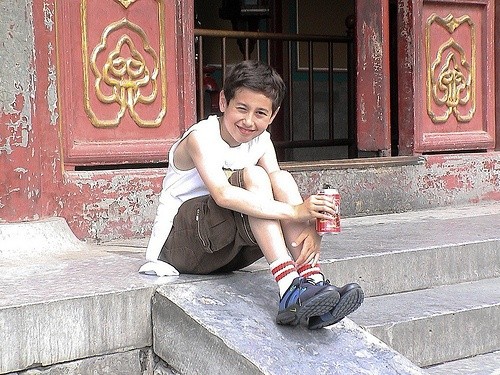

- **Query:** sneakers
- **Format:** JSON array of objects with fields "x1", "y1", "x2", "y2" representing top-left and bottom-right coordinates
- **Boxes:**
[
  {"x1": 306, "y1": 279, "x2": 364, "y2": 329},
  {"x1": 276, "y1": 276, "x2": 340, "y2": 327}
]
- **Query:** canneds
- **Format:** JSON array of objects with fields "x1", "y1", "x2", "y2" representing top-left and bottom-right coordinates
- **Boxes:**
[{"x1": 315, "y1": 189, "x2": 341, "y2": 236}]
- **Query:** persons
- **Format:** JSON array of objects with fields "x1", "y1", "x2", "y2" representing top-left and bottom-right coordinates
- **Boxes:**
[{"x1": 144, "y1": 60, "x2": 364, "y2": 330}]
